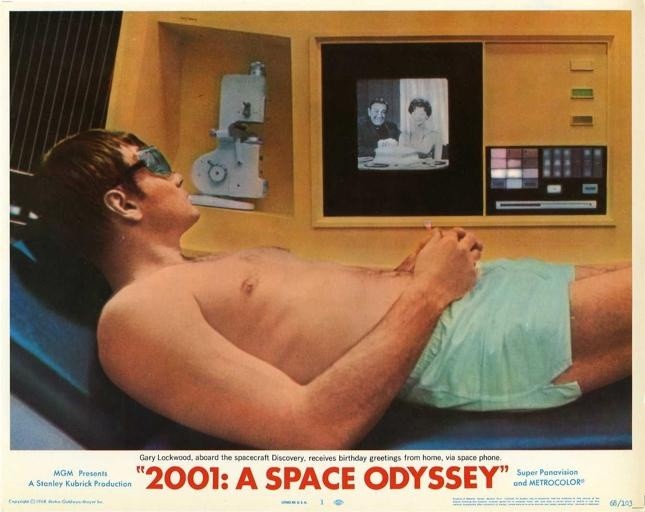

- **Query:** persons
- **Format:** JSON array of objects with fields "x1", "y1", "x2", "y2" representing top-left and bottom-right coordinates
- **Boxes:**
[
  {"x1": 30, "y1": 127, "x2": 631, "y2": 450},
  {"x1": 397, "y1": 97, "x2": 443, "y2": 162},
  {"x1": 355, "y1": 93, "x2": 402, "y2": 156}
]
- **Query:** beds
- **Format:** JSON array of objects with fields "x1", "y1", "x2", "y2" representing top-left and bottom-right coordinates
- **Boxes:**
[{"x1": 11, "y1": 239, "x2": 631, "y2": 448}]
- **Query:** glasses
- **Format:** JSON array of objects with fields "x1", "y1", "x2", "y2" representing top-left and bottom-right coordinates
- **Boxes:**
[{"x1": 112, "y1": 144, "x2": 172, "y2": 188}]
